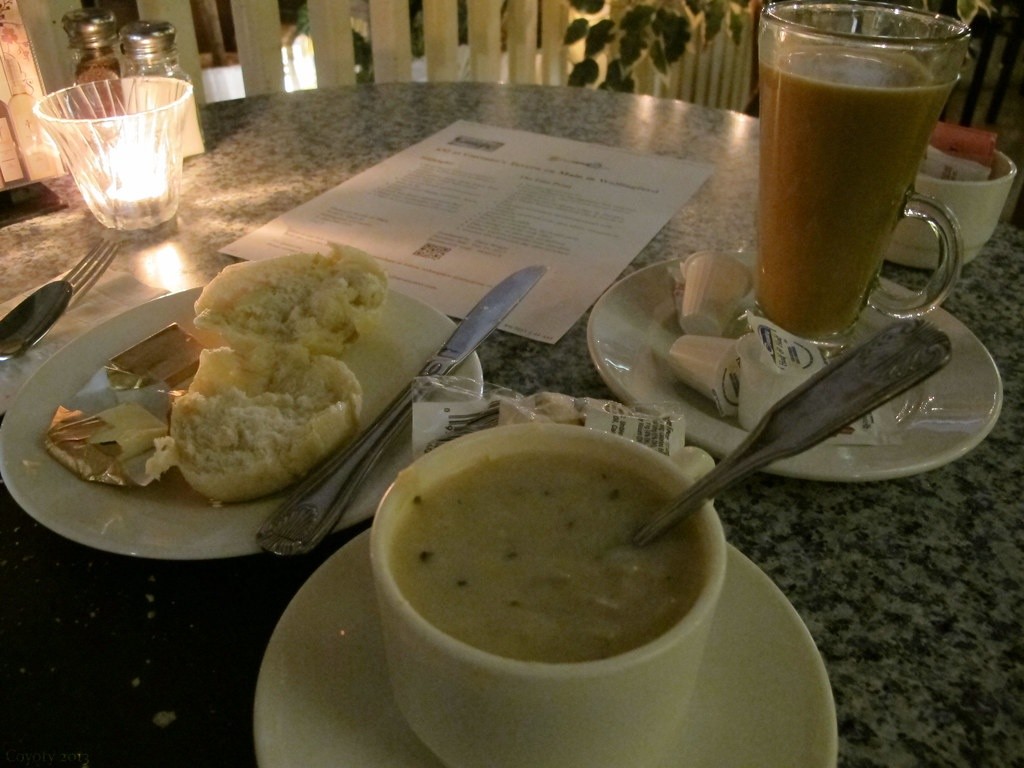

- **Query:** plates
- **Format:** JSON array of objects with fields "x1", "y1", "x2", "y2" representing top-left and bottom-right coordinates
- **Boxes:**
[
  {"x1": 0, "y1": 278, "x2": 487, "y2": 558},
  {"x1": 586, "y1": 250, "x2": 1004, "y2": 480},
  {"x1": 251, "y1": 505, "x2": 840, "y2": 768}
]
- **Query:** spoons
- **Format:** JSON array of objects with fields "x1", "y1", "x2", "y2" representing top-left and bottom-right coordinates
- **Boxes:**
[{"x1": 0, "y1": 280, "x2": 72, "y2": 366}]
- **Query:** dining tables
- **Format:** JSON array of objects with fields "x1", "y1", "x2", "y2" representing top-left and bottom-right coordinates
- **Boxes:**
[{"x1": 0, "y1": 81, "x2": 1024, "y2": 767}]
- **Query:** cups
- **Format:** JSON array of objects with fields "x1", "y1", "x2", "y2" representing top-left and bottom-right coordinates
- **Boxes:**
[
  {"x1": 745, "y1": 3, "x2": 972, "y2": 354},
  {"x1": 878, "y1": 146, "x2": 1018, "y2": 268},
  {"x1": 369, "y1": 424, "x2": 728, "y2": 768}
]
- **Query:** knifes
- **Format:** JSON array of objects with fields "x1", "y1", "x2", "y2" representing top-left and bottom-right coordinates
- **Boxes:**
[{"x1": 255, "y1": 263, "x2": 549, "y2": 556}]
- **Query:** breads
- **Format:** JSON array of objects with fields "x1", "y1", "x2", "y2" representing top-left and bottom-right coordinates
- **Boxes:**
[
  {"x1": 170, "y1": 235, "x2": 389, "y2": 358},
  {"x1": 175, "y1": 340, "x2": 361, "y2": 497}
]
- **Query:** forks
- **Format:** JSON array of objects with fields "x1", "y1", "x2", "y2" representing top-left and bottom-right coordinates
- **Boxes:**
[{"x1": 61, "y1": 239, "x2": 123, "y2": 310}]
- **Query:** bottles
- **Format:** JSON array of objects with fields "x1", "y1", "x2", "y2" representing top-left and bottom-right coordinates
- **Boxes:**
[
  {"x1": 62, "y1": 11, "x2": 120, "y2": 86},
  {"x1": 119, "y1": 20, "x2": 207, "y2": 159}
]
